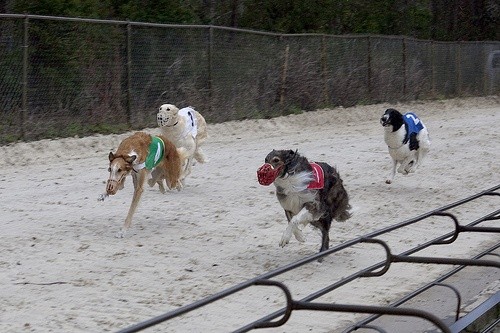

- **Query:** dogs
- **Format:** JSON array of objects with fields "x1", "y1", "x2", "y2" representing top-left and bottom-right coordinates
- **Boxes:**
[
  {"x1": 264, "y1": 149, "x2": 354, "y2": 263},
  {"x1": 380, "y1": 108, "x2": 430, "y2": 184},
  {"x1": 157, "y1": 104, "x2": 208, "y2": 182},
  {"x1": 97, "y1": 131, "x2": 181, "y2": 239}
]
